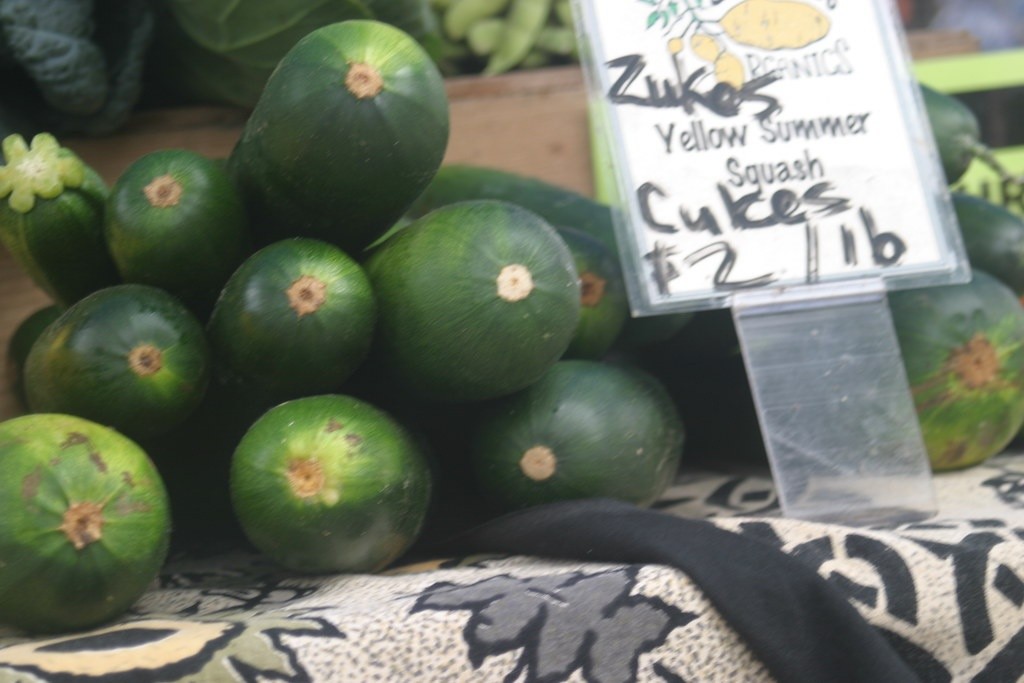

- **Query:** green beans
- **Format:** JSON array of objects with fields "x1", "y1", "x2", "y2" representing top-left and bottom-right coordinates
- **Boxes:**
[{"x1": 442, "y1": 0, "x2": 585, "y2": 83}]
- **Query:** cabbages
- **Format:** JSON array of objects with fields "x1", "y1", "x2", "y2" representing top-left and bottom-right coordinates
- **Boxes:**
[{"x1": 0, "y1": 0, "x2": 442, "y2": 137}]
